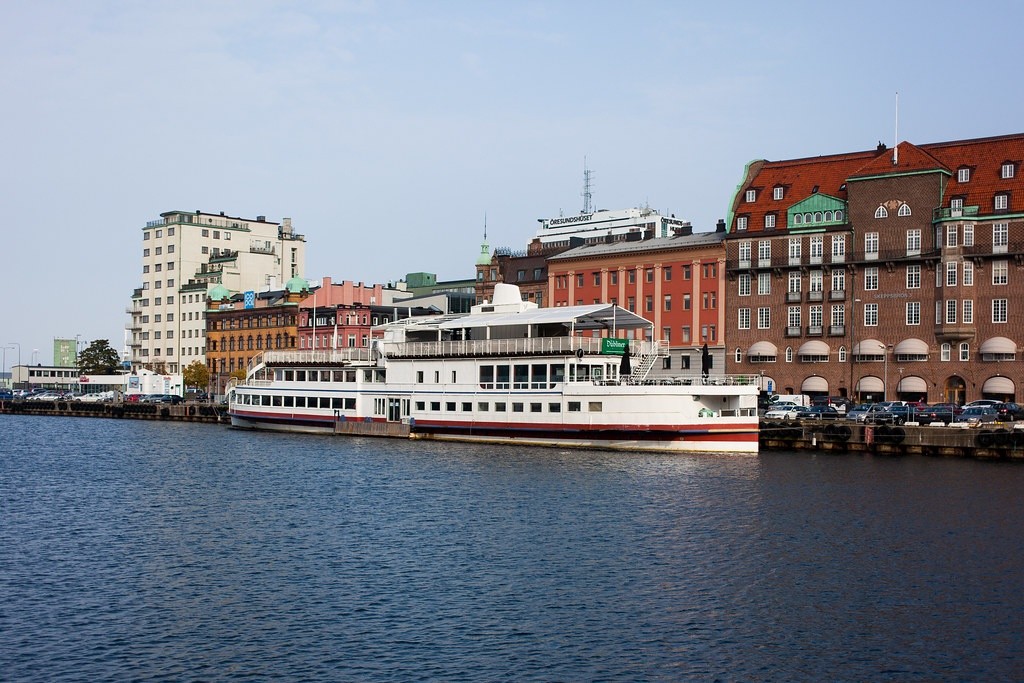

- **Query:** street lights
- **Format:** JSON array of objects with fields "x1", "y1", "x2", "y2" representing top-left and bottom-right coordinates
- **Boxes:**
[
  {"x1": 854, "y1": 297, "x2": 863, "y2": 404},
  {"x1": 878, "y1": 344, "x2": 895, "y2": 405},
  {"x1": 8, "y1": 342, "x2": 21, "y2": 387},
  {"x1": 0, "y1": 346, "x2": 16, "y2": 387}
]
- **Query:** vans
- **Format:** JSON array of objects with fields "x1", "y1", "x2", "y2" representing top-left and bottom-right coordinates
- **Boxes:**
[{"x1": 764, "y1": 394, "x2": 811, "y2": 408}]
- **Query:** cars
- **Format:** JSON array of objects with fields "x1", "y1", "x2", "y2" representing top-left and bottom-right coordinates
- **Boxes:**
[
  {"x1": 220, "y1": 400, "x2": 229, "y2": 405},
  {"x1": 955, "y1": 406, "x2": 999, "y2": 427},
  {"x1": 186, "y1": 388, "x2": 202, "y2": 394},
  {"x1": 796, "y1": 406, "x2": 840, "y2": 422},
  {"x1": 197, "y1": 392, "x2": 213, "y2": 401},
  {"x1": 875, "y1": 406, "x2": 914, "y2": 427},
  {"x1": 878, "y1": 400, "x2": 906, "y2": 409},
  {"x1": 905, "y1": 402, "x2": 930, "y2": 412},
  {"x1": 991, "y1": 402, "x2": 1024, "y2": 422},
  {"x1": 911, "y1": 406, "x2": 957, "y2": 424},
  {"x1": 764, "y1": 405, "x2": 809, "y2": 422},
  {"x1": 845, "y1": 402, "x2": 881, "y2": 425},
  {"x1": 929, "y1": 402, "x2": 962, "y2": 414},
  {"x1": 768, "y1": 401, "x2": 797, "y2": 412},
  {"x1": 0, "y1": 386, "x2": 188, "y2": 407}
]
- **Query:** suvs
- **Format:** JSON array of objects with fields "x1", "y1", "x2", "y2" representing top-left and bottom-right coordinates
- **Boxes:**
[
  {"x1": 814, "y1": 396, "x2": 857, "y2": 412},
  {"x1": 960, "y1": 400, "x2": 1003, "y2": 411}
]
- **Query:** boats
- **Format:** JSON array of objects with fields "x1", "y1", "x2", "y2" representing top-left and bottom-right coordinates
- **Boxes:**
[{"x1": 230, "y1": 282, "x2": 763, "y2": 453}]
